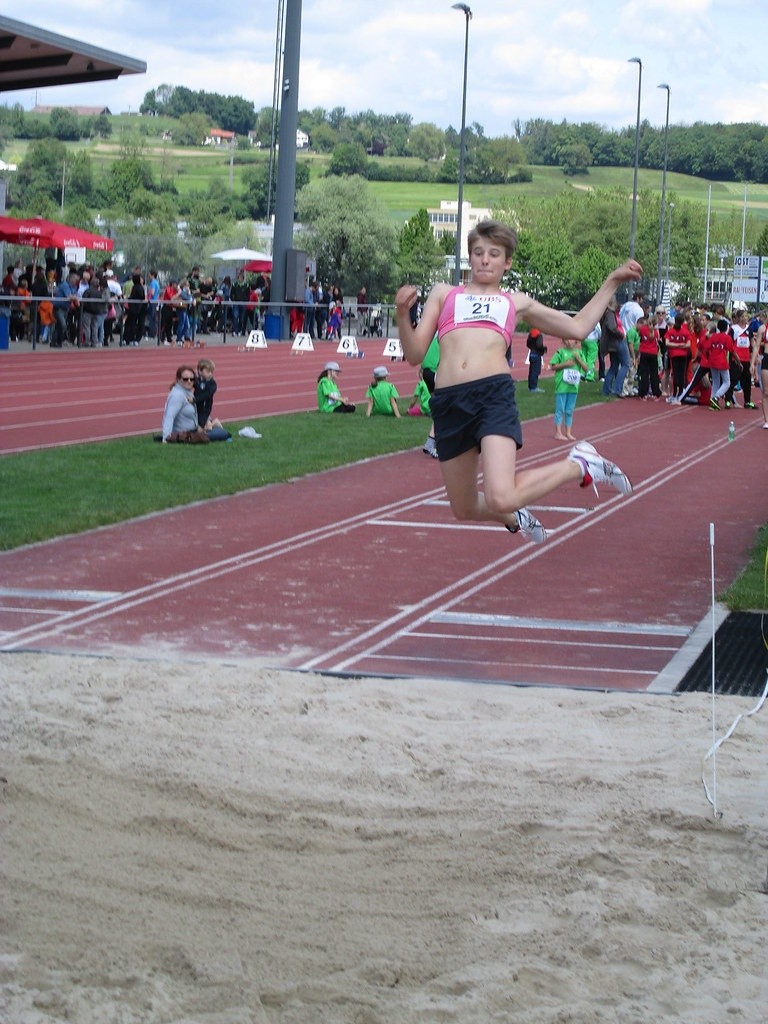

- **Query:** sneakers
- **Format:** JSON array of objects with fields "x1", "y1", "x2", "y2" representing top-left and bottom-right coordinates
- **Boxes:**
[
  {"x1": 724, "y1": 401, "x2": 730, "y2": 408},
  {"x1": 744, "y1": 402, "x2": 760, "y2": 409},
  {"x1": 567, "y1": 441, "x2": 634, "y2": 497},
  {"x1": 708, "y1": 406, "x2": 718, "y2": 411},
  {"x1": 710, "y1": 398, "x2": 721, "y2": 410},
  {"x1": 503, "y1": 507, "x2": 548, "y2": 544}
]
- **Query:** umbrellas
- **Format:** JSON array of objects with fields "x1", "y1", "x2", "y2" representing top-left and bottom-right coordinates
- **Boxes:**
[
  {"x1": 210, "y1": 246, "x2": 272, "y2": 284},
  {"x1": 0, "y1": 214, "x2": 115, "y2": 284},
  {"x1": 242, "y1": 260, "x2": 310, "y2": 272}
]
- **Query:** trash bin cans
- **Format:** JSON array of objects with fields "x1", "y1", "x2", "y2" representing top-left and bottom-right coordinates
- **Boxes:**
[
  {"x1": 265, "y1": 313, "x2": 284, "y2": 339},
  {"x1": 0, "y1": 308, "x2": 11, "y2": 349}
]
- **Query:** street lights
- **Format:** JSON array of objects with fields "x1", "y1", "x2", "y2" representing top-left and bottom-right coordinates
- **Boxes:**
[
  {"x1": 453, "y1": 0, "x2": 470, "y2": 291},
  {"x1": 629, "y1": 52, "x2": 642, "y2": 278},
  {"x1": 656, "y1": 84, "x2": 672, "y2": 307}
]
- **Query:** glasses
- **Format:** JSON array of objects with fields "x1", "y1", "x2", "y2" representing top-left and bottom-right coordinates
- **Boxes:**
[
  {"x1": 182, "y1": 376, "x2": 195, "y2": 382},
  {"x1": 656, "y1": 311, "x2": 666, "y2": 316}
]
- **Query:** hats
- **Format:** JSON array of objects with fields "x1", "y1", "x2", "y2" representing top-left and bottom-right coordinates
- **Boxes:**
[
  {"x1": 324, "y1": 362, "x2": 342, "y2": 372},
  {"x1": 373, "y1": 365, "x2": 389, "y2": 378}
]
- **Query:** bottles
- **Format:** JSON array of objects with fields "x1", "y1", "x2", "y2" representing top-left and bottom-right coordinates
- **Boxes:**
[{"x1": 728, "y1": 422, "x2": 735, "y2": 441}]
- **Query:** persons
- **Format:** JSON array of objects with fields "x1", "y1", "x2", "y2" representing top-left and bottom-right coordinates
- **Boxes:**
[
  {"x1": 394, "y1": 222, "x2": 645, "y2": 541},
  {"x1": 639, "y1": 304, "x2": 768, "y2": 431},
  {"x1": 408, "y1": 367, "x2": 432, "y2": 418},
  {"x1": 365, "y1": 367, "x2": 402, "y2": 419},
  {"x1": 162, "y1": 360, "x2": 230, "y2": 445},
  {"x1": 316, "y1": 362, "x2": 356, "y2": 413},
  {"x1": 2, "y1": 258, "x2": 371, "y2": 346}
]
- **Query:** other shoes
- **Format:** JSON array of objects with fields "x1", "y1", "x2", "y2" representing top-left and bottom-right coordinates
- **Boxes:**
[
  {"x1": 762, "y1": 422, "x2": 768, "y2": 429},
  {"x1": 733, "y1": 403, "x2": 743, "y2": 408},
  {"x1": 580, "y1": 375, "x2": 596, "y2": 384},
  {"x1": 530, "y1": 387, "x2": 546, "y2": 393},
  {"x1": 566, "y1": 434, "x2": 576, "y2": 440},
  {"x1": 554, "y1": 433, "x2": 570, "y2": 441},
  {"x1": 615, "y1": 392, "x2": 625, "y2": 398},
  {"x1": 654, "y1": 395, "x2": 661, "y2": 402},
  {"x1": 599, "y1": 377, "x2": 605, "y2": 382},
  {"x1": 666, "y1": 396, "x2": 676, "y2": 403},
  {"x1": 671, "y1": 400, "x2": 681, "y2": 405},
  {"x1": 36, "y1": 338, "x2": 202, "y2": 349},
  {"x1": 640, "y1": 395, "x2": 647, "y2": 401}
]
